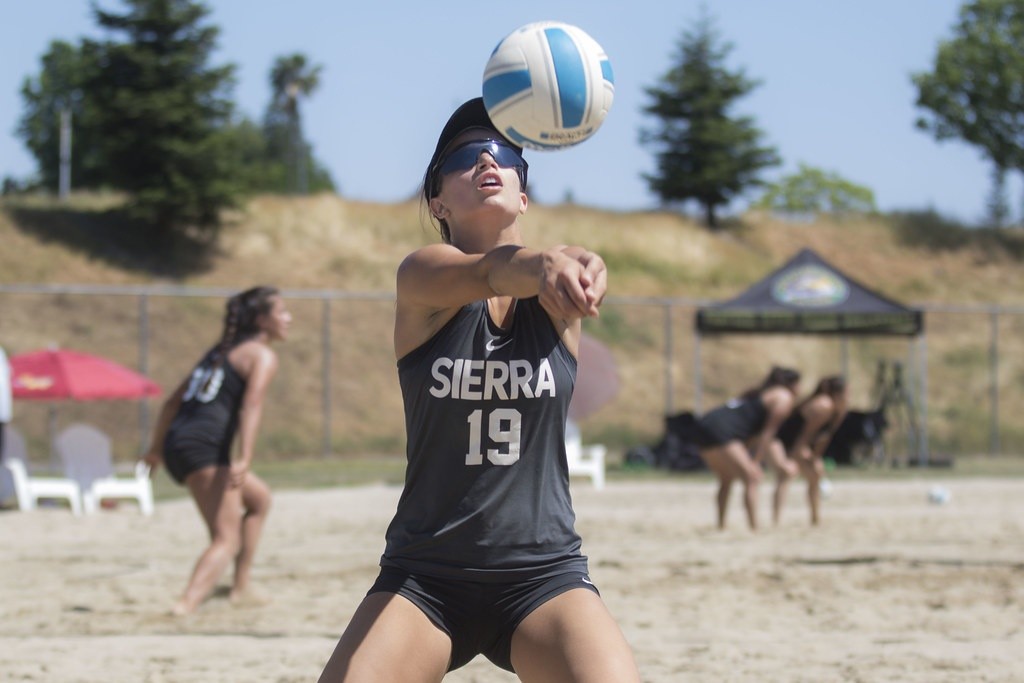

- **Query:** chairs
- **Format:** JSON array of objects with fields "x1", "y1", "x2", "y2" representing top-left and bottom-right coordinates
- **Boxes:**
[
  {"x1": 562, "y1": 414, "x2": 610, "y2": 490},
  {"x1": 52, "y1": 419, "x2": 116, "y2": 492},
  {"x1": 5, "y1": 459, "x2": 83, "y2": 518},
  {"x1": 88, "y1": 455, "x2": 152, "y2": 518}
]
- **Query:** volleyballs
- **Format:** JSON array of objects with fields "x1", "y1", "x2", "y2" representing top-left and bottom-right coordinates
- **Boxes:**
[{"x1": 481, "y1": 20, "x2": 615, "y2": 151}]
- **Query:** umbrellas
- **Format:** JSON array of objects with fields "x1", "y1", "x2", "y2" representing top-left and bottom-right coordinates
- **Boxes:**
[{"x1": 1, "y1": 341, "x2": 166, "y2": 402}]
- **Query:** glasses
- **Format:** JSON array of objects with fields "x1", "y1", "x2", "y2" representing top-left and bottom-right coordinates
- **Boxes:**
[{"x1": 432, "y1": 140, "x2": 528, "y2": 188}]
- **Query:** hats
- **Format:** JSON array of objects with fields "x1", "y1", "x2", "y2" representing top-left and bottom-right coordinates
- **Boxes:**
[{"x1": 428, "y1": 96, "x2": 523, "y2": 163}]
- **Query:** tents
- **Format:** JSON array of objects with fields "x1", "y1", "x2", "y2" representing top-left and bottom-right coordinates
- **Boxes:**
[{"x1": 690, "y1": 248, "x2": 929, "y2": 469}]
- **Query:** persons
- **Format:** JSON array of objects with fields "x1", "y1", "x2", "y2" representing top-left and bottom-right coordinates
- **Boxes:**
[
  {"x1": 773, "y1": 375, "x2": 851, "y2": 527},
  {"x1": 142, "y1": 284, "x2": 293, "y2": 621},
  {"x1": 687, "y1": 365, "x2": 801, "y2": 529},
  {"x1": 313, "y1": 120, "x2": 633, "y2": 683}
]
